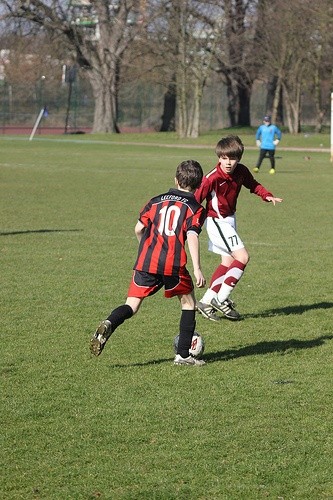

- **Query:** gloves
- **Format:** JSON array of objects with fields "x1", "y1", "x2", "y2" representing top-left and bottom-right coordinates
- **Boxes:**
[
  {"x1": 274, "y1": 139, "x2": 279, "y2": 145},
  {"x1": 256, "y1": 140, "x2": 260, "y2": 146}
]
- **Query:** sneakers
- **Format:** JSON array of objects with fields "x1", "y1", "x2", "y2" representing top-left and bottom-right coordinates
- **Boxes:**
[
  {"x1": 90, "y1": 319, "x2": 111, "y2": 357},
  {"x1": 173, "y1": 353, "x2": 205, "y2": 367},
  {"x1": 196, "y1": 302, "x2": 220, "y2": 323},
  {"x1": 210, "y1": 296, "x2": 239, "y2": 319}
]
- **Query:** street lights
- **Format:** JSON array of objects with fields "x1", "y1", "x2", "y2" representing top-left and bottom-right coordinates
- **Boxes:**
[{"x1": 39, "y1": 75, "x2": 46, "y2": 109}]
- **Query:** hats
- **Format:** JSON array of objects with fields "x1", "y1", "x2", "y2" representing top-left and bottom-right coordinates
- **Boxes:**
[{"x1": 263, "y1": 116, "x2": 270, "y2": 121}]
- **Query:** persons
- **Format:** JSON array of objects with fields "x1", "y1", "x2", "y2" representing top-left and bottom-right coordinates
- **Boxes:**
[
  {"x1": 194, "y1": 135, "x2": 283, "y2": 323},
  {"x1": 89, "y1": 160, "x2": 206, "y2": 367},
  {"x1": 252, "y1": 116, "x2": 281, "y2": 176}
]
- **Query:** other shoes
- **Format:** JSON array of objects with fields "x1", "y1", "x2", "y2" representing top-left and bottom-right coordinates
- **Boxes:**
[
  {"x1": 254, "y1": 167, "x2": 259, "y2": 172},
  {"x1": 269, "y1": 168, "x2": 275, "y2": 174}
]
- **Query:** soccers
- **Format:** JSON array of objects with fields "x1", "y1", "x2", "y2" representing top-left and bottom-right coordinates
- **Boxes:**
[{"x1": 174, "y1": 330, "x2": 205, "y2": 359}]
